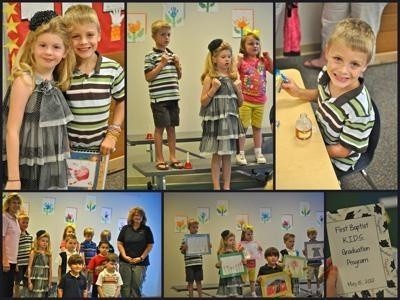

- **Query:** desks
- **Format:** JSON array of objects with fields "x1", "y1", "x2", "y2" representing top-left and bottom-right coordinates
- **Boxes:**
[{"x1": 276, "y1": 69, "x2": 342, "y2": 190}]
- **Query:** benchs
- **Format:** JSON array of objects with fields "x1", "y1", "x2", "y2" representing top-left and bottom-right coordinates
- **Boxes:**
[
  {"x1": 127, "y1": 125, "x2": 273, "y2": 191},
  {"x1": 170, "y1": 277, "x2": 323, "y2": 297},
  {"x1": 130, "y1": 152, "x2": 272, "y2": 191}
]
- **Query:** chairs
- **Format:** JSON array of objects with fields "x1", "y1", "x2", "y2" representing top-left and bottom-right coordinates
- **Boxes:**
[{"x1": 336, "y1": 95, "x2": 381, "y2": 190}]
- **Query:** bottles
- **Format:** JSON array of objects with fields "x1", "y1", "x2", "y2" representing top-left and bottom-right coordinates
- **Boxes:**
[{"x1": 294, "y1": 112, "x2": 313, "y2": 142}]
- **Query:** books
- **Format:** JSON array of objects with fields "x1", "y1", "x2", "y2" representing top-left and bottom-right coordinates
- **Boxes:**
[{"x1": 65, "y1": 148, "x2": 109, "y2": 190}]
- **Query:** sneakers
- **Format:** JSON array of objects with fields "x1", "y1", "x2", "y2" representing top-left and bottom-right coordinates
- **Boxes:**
[
  {"x1": 236, "y1": 154, "x2": 247, "y2": 166},
  {"x1": 255, "y1": 153, "x2": 266, "y2": 164}
]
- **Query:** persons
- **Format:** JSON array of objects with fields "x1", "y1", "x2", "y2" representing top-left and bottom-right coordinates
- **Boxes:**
[
  {"x1": 236, "y1": 30, "x2": 274, "y2": 165},
  {"x1": 144, "y1": 20, "x2": 183, "y2": 170},
  {"x1": 1, "y1": 10, "x2": 74, "y2": 191},
  {"x1": 2, "y1": 193, "x2": 154, "y2": 300},
  {"x1": 200, "y1": 39, "x2": 246, "y2": 191},
  {"x1": 59, "y1": 5, "x2": 125, "y2": 155},
  {"x1": 180, "y1": 218, "x2": 338, "y2": 299},
  {"x1": 277, "y1": 17, "x2": 375, "y2": 188},
  {"x1": 303, "y1": 2, "x2": 388, "y2": 69}
]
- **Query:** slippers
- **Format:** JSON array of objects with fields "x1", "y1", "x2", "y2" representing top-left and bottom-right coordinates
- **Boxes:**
[
  {"x1": 170, "y1": 161, "x2": 183, "y2": 169},
  {"x1": 156, "y1": 162, "x2": 169, "y2": 170}
]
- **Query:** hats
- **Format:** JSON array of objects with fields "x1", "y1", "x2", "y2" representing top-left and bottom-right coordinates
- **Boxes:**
[
  {"x1": 30, "y1": 11, "x2": 56, "y2": 31},
  {"x1": 221, "y1": 231, "x2": 229, "y2": 239},
  {"x1": 36, "y1": 230, "x2": 45, "y2": 241},
  {"x1": 208, "y1": 39, "x2": 222, "y2": 51}
]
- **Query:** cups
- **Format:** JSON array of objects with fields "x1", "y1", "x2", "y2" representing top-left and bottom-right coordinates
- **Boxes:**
[{"x1": 147, "y1": 133, "x2": 153, "y2": 139}]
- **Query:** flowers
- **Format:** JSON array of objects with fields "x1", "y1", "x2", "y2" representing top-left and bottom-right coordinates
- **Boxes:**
[
  {"x1": 18, "y1": 198, "x2": 126, "y2": 231},
  {"x1": 127, "y1": 1, "x2": 252, "y2": 43},
  {"x1": 174, "y1": 201, "x2": 324, "y2": 231}
]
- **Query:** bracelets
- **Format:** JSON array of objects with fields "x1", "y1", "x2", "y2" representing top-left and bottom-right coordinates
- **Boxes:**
[
  {"x1": 7, "y1": 179, "x2": 21, "y2": 181},
  {"x1": 206, "y1": 93, "x2": 214, "y2": 98},
  {"x1": 105, "y1": 121, "x2": 121, "y2": 140}
]
